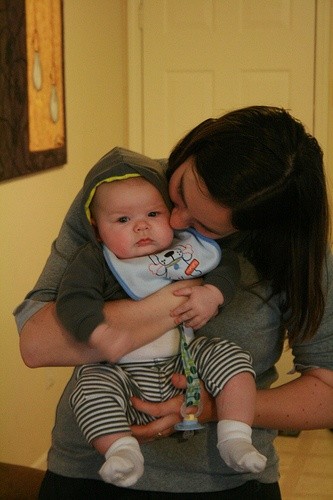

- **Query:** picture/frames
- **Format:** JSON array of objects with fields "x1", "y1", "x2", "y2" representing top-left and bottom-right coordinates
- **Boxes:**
[{"x1": 0, "y1": 0, "x2": 68, "y2": 185}]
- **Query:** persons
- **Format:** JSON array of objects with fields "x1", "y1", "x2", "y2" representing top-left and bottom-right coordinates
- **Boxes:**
[
  {"x1": 11, "y1": 105, "x2": 332, "y2": 496},
  {"x1": 58, "y1": 144, "x2": 267, "y2": 487}
]
